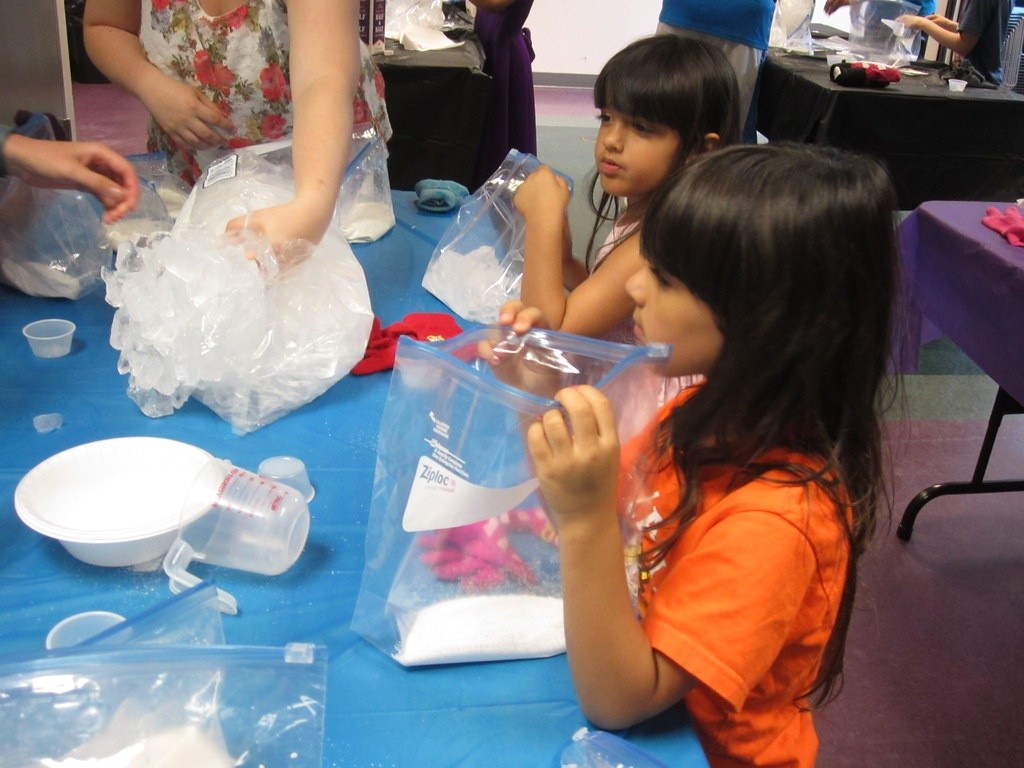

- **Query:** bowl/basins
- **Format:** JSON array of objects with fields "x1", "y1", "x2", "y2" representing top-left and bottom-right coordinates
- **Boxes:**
[{"x1": 12, "y1": 433, "x2": 225, "y2": 569}]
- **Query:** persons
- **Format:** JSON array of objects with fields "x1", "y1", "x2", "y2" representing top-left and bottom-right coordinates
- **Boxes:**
[
  {"x1": 514, "y1": 32, "x2": 742, "y2": 345},
  {"x1": 895, "y1": 0, "x2": 1012, "y2": 85},
  {"x1": 0, "y1": 131, "x2": 138, "y2": 224},
  {"x1": 477, "y1": 141, "x2": 901, "y2": 767},
  {"x1": 84, "y1": 0, "x2": 394, "y2": 272},
  {"x1": 659, "y1": 1, "x2": 778, "y2": 155},
  {"x1": 466, "y1": 0, "x2": 538, "y2": 168},
  {"x1": 823, "y1": 0, "x2": 933, "y2": 61}
]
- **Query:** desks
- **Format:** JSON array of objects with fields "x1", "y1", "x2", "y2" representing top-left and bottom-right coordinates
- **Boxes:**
[
  {"x1": 890, "y1": 200, "x2": 1024, "y2": 540},
  {"x1": 764, "y1": 47, "x2": 1024, "y2": 210},
  {"x1": 0, "y1": 184, "x2": 714, "y2": 768},
  {"x1": 363, "y1": 30, "x2": 494, "y2": 191}
]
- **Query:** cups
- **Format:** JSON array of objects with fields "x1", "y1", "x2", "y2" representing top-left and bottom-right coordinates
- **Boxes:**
[
  {"x1": 45, "y1": 609, "x2": 130, "y2": 650},
  {"x1": 949, "y1": 79, "x2": 968, "y2": 93},
  {"x1": 22, "y1": 318, "x2": 76, "y2": 359},
  {"x1": 257, "y1": 456, "x2": 316, "y2": 505}
]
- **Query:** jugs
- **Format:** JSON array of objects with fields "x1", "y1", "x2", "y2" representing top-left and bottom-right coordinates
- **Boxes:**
[{"x1": 168, "y1": 456, "x2": 314, "y2": 618}]
